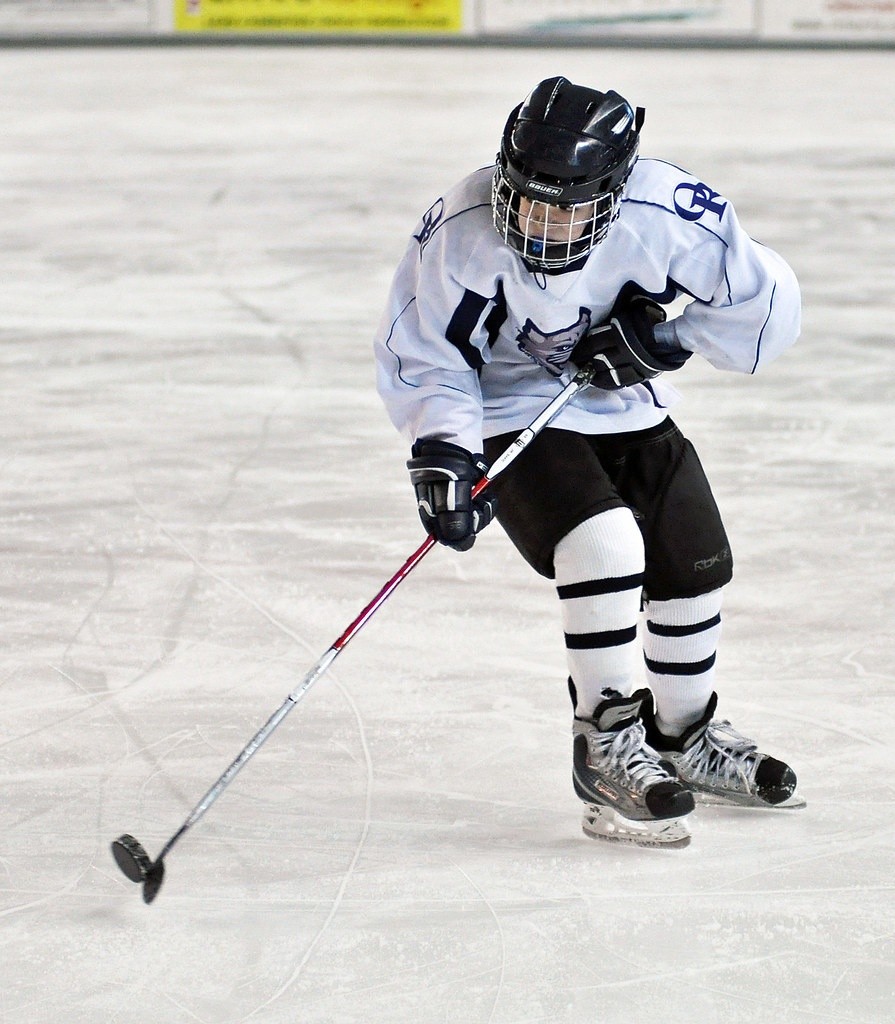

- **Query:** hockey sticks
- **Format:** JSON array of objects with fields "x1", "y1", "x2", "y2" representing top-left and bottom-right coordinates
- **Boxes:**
[{"x1": 140, "y1": 296, "x2": 668, "y2": 905}]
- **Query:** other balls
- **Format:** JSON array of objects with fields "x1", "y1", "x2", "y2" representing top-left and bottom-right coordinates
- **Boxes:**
[{"x1": 110, "y1": 831, "x2": 154, "y2": 885}]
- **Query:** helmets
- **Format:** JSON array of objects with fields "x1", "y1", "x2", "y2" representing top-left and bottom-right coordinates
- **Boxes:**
[{"x1": 491, "y1": 76, "x2": 646, "y2": 276}]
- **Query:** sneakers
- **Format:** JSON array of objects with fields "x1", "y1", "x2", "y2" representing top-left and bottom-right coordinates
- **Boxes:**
[
  {"x1": 568, "y1": 676, "x2": 694, "y2": 847},
  {"x1": 640, "y1": 688, "x2": 806, "y2": 808}
]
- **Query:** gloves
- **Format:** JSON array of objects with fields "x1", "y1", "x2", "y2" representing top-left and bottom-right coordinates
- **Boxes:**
[
  {"x1": 408, "y1": 438, "x2": 499, "y2": 551},
  {"x1": 575, "y1": 295, "x2": 694, "y2": 391}
]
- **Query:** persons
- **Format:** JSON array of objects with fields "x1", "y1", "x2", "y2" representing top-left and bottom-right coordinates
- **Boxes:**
[{"x1": 372, "y1": 76, "x2": 807, "y2": 847}]
